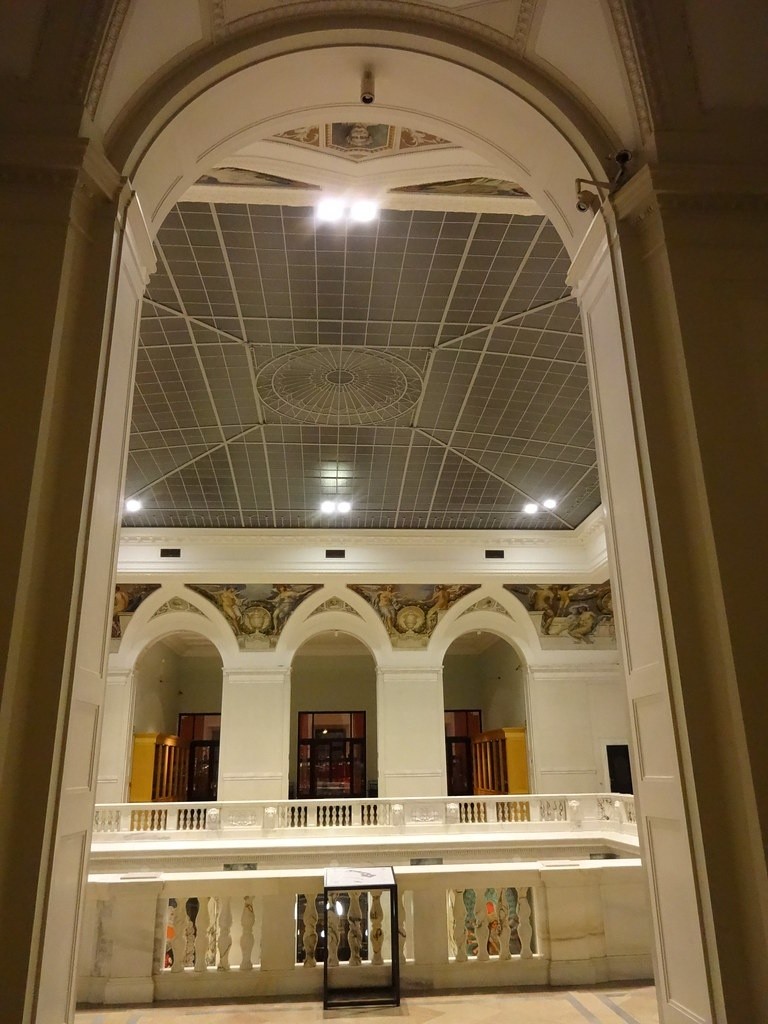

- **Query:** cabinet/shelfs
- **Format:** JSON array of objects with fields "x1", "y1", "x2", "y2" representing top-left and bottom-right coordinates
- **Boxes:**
[
  {"x1": 128, "y1": 732, "x2": 181, "y2": 814},
  {"x1": 473, "y1": 727, "x2": 529, "y2": 813}
]
